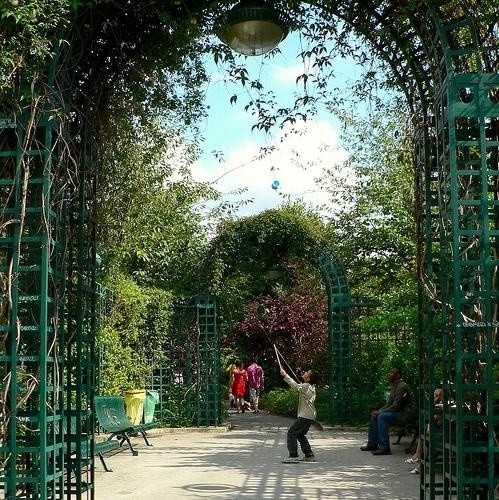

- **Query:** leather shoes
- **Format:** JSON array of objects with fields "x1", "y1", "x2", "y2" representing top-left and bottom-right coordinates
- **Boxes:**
[
  {"x1": 360, "y1": 446, "x2": 379, "y2": 451},
  {"x1": 372, "y1": 449, "x2": 392, "y2": 455}
]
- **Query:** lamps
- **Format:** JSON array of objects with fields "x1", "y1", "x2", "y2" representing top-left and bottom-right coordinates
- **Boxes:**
[{"x1": 213, "y1": 0, "x2": 291, "y2": 59}]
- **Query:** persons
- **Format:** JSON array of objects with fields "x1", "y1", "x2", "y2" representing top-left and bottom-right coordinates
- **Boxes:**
[
  {"x1": 246, "y1": 359, "x2": 265, "y2": 414},
  {"x1": 405, "y1": 388, "x2": 443, "y2": 474},
  {"x1": 280, "y1": 367, "x2": 319, "y2": 464},
  {"x1": 360, "y1": 366, "x2": 418, "y2": 455},
  {"x1": 231, "y1": 360, "x2": 249, "y2": 414}
]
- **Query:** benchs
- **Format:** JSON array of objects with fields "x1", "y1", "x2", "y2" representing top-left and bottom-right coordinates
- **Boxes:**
[
  {"x1": 51, "y1": 407, "x2": 118, "y2": 472},
  {"x1": 388, "y1": 423, "x2": 417, "y2": 454},
  {"x1": 92, "y1": 395, "x2": 158, "y2": 456}
]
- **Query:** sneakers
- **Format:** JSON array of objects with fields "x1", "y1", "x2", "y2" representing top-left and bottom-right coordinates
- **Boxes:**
[
  {"x1": 300, "y1": 453, "x2": 316, "y2": 462},
  {"x1": 404, "y1": 458, "x2": 419, "y2": 463},
  {"x1": 229, "y1": 399, "x2": 259, "y2": 415},
  {"x1": 281, "y1": 456, "x2": 300, "y2": 464},
  {"x1": 410, "y1": 468, "x2": 419, "y2": 474}
]
